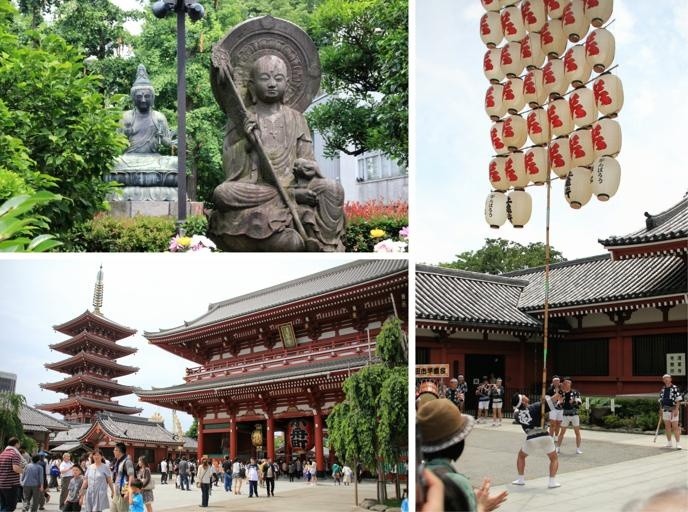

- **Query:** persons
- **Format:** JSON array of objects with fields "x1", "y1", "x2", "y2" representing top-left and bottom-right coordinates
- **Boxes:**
[
  {"x1": 511, "y1": 385, "x2": 562, "y2": 488},
  {"x1": 556, "y1": 377, "x2": 583, "y2": 454},
  {"x1": 0, "y1": 437, "x2": 155, "y2": 512},
  {"x1": 545, "y1": 375, "x2": 564, "y2": 442},
  {"x1": 114, "y1": 63, "x2": 172, "y2": 154},
  {"x1": 160, "y1": 451, "x2": 353, "y2": 508},
  {"x1": 212, "y1": 52, "x2": 346, "y2": 251},
  {"x1": 657, "y1": 372, "x2": 684, "y2": 450},
  {"x1": 415, "y1": 375, "x2": 509, "y2": 511}
]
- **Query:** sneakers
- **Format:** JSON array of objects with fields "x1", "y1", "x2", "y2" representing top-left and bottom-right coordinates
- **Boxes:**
[
  {"x1": 198, "y1": 504, "x2": 209, "y2": 508},
  {"x1": 555, "y1": 447, "x2": 559, "y2": 453},
  {"x1": 664, "y1": 441, "x2": 672, "y2": 447},
  {"x1": 39, "y1": 488, "x2": 59, "y2": 511},
  {"x1": 160, "y1": 482, "x2": 275, "y2": 498},
  {"x1": 675, "y1": 443, "x2": 681, "y2": 450},
  {"x1": 511, "y1": 478, "x2": 526, "y2": 485},
  {"x1": 575, "y1": 448, "x2": 582, "y2": 454},
  {"x1": 546, "y1": 478, "x2": 559, "y2": 487}
]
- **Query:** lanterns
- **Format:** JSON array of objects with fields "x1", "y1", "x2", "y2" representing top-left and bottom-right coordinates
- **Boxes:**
[
  {"x1": 481, "y1": 1, "x2": 630, "y2": 155},
  {"x1": 562, "y1": 168, "x2": 590, "y2": 208},
  {"x1": 489, "y1": 157, "x2": 511, "y2": 192},
  {"x1": 569, "y1": 129, "x2": 592, "y2": 167},
  {"x1": 506, "y1": 189, "x2": 532, "y2": 228},
  {"x1": 590, "y1": 156, "x2": 621, "y2": 201},
  {"x1": 485, "y1": 190, "x2": 506, "y2": 229},
  {"x1": 505, "y1": 149, "x2": 531, "y2": 190},
  {"x1": 526, "y1": 148, "x2": 549, "y2": 185},
  {"x1": 549, "y1": 140, "x2": 570, "y2": 177},
  {"x1": 286, "y1": 418, "x2": 315, "y2": 454}
]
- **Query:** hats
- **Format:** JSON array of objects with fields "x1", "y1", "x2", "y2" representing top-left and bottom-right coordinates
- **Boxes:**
[{"x1": 417, "y1": 398, "x2": 475, "y2": 454}]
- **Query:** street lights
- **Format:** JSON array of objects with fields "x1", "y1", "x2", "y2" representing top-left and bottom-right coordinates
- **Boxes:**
[{"x1": 152, "y1": 0, "x2": 205, "y2": 222}]
